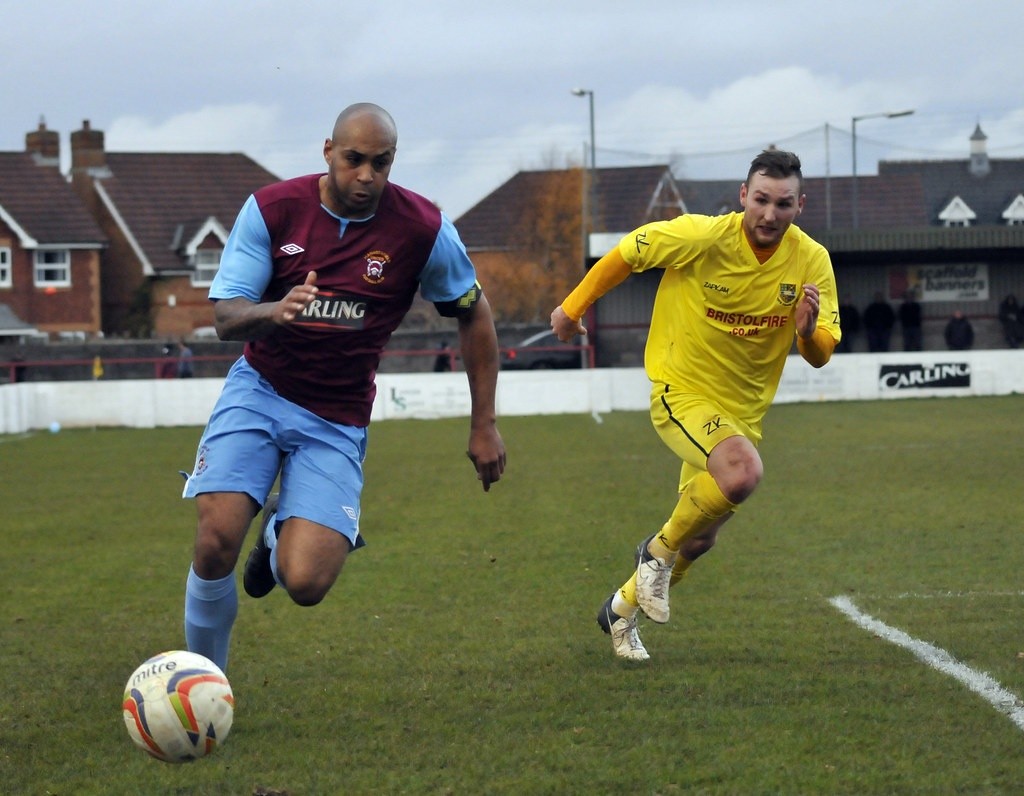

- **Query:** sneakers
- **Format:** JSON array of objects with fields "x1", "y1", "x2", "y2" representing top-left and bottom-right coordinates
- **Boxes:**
[
  {"x1": 634, "y1": 534, "x2": 676, "y2": 625},
  {"x1": 598, "y1": 592, "x2": 650, "y2": 662},
  {"x1": 242, "y1": 493, "x2": 277, "y2": 598}
]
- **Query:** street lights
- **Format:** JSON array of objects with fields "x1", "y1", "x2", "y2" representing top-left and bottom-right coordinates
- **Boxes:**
[
  {"x1": 569, "y1": 89, "x2": 601, "y2": 232},
  {"x1": 851, "y1": 108, "x2": 917, "y2": 229}
]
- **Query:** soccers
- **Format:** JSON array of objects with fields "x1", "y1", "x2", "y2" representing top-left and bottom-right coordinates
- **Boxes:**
[{"x1": 122, "y1": 649, "x2": 235, "y2": 763}]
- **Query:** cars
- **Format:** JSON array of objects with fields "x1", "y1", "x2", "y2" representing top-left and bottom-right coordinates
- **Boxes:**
[{"x1": 498, "y1": 326, "x2": 619, "y2": 371}]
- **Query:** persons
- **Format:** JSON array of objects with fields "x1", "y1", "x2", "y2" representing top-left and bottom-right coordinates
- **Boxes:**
[
  {"x1": 863, "y1": 292, "x2": 894, "y2": 352},
  {"x1": 945, "y1": 310, "x2": 974, "y2": 349},
  {"x1": 183, "y1": 103, "x2": 507, "y2": 671},
  {"x1": 894, "y1": 295, "x2": 922, "y2": 351},
  {"x1": 550, "y1": 151, "x2": 843, "y2": 661},
  {"x1": 434, "y1": 342, "x2": 450, "y2": 371},
  {"x1": 999, "y1": 295, "x2": 1024, "y2": 348},
  {"x1": 178, "y1": 342, "x2": 193, "y2": 378},
  {"x1": 839, "y1": 293, "x2": 863, "y2": 353}
]
